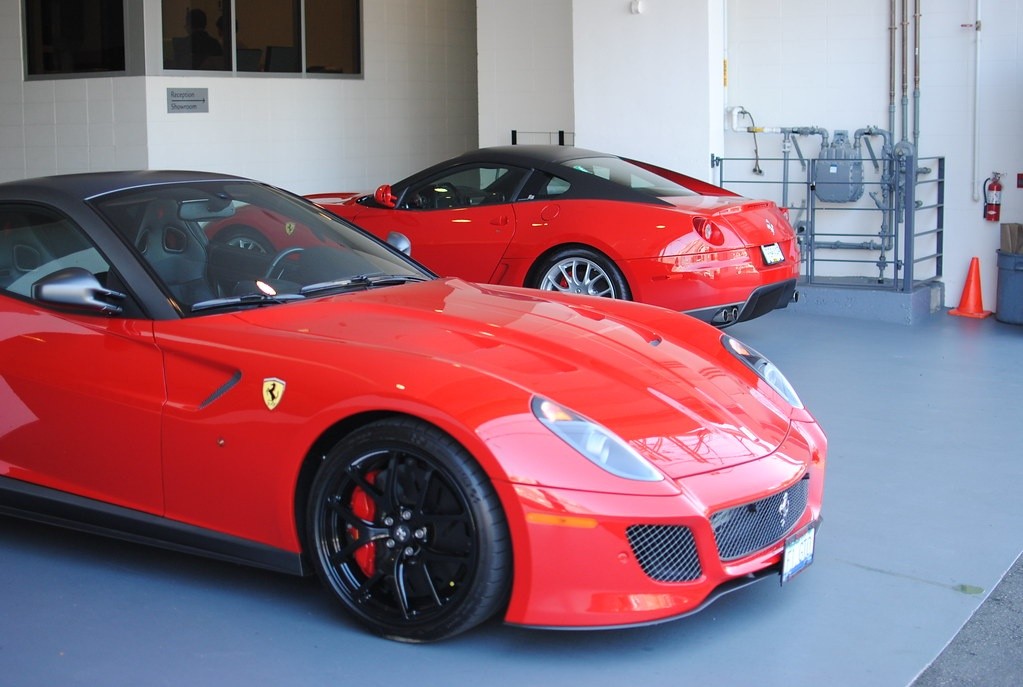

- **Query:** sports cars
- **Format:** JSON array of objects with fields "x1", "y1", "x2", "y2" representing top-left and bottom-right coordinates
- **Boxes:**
[
  {"x1": 1, "y1": 168, "x2": 833, "y2": 649},
  {"x1": 201, "y1": 143, "x2": 803, "y2": 332}
]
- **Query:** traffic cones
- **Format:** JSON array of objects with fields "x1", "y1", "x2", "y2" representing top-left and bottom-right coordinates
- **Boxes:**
[{"x1": 945, "y1": 255, "x2": 993, "y2": 320}]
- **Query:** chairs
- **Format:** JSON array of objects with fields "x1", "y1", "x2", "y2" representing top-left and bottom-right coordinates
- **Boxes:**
[
  {"x1": 477, "y1": 171, "x2": 549, "y2": 208},
  {"x1": 110, "y1": 194, "x2": 218, "y2": 318},
  {"x1": 0, "y1": 208, "x2": 67, "y2": 301}
]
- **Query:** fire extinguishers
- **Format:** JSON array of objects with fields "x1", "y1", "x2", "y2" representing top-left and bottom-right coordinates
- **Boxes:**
[{"x1": 982, "y1": 171, "x2": 1007, "y2": 222}]
